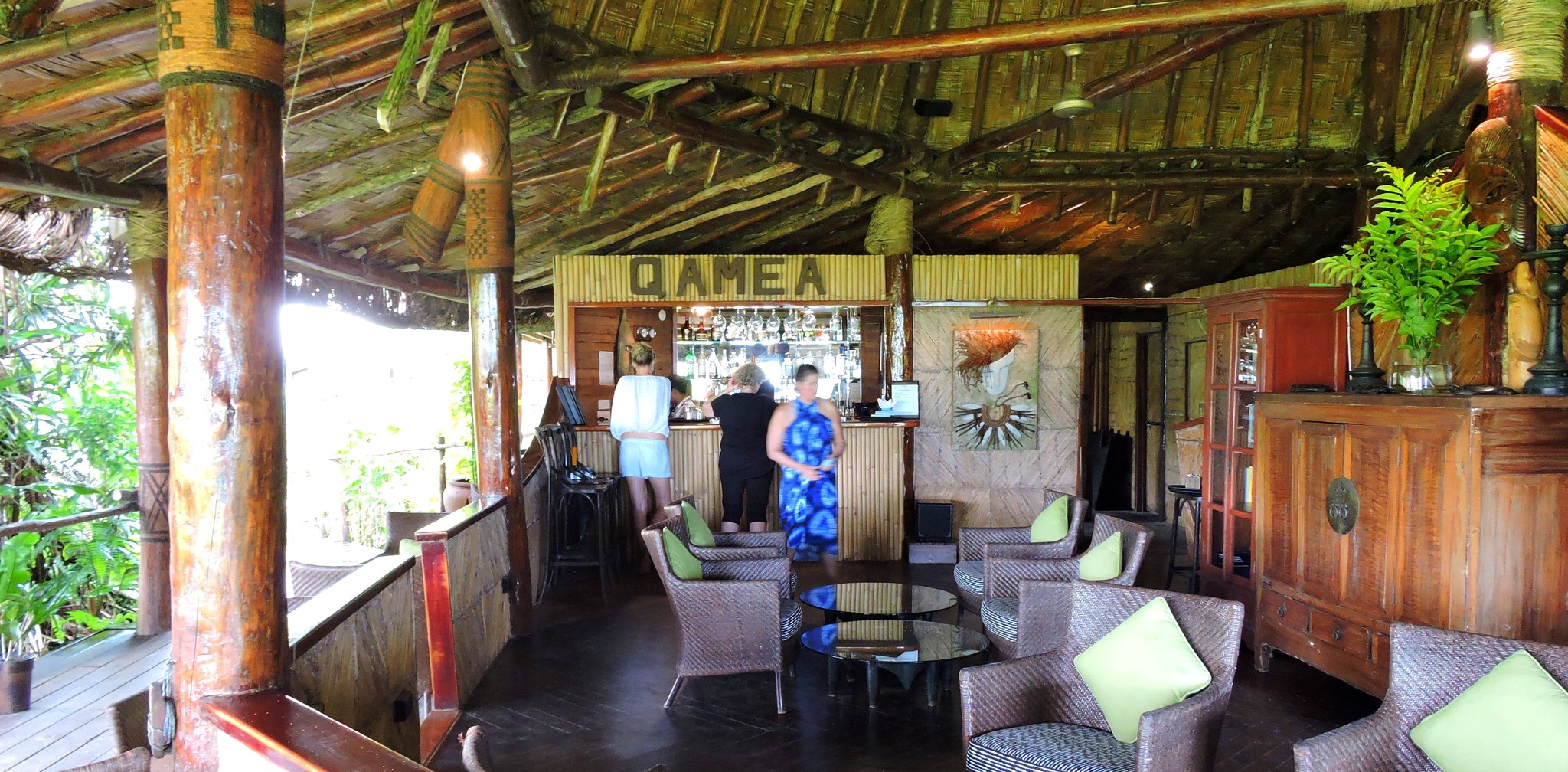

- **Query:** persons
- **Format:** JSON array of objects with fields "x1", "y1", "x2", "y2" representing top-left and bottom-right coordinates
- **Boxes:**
[
  {"x1": 765, "y1": 364, "x2": 846, "y2": 577},
  {"x1": 610, "y1": 341, "x2": 672, "y2": 584},
  {"x1": 667, "y1": 374, "x2": 696, "y2": 419},
  {"x1": 701, "y1": 362, "x2": 779, "y2": 534}
]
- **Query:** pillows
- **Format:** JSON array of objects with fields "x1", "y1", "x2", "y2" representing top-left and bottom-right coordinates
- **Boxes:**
[
  {"x1": 1079, "y1": 531, "x2": 1123, "y2": 581},
  {"x1": 399, "y1": 539, "x2": 422, "y2": 557},
  {"x1": 1073, "y1": 596, "x2": 1212, "y2": 743},
  {"x1": 1031, "y1": 495, "x2": 1068, "y2": 543},
  {"x1": 681, "y1": 500, "x2": 716, "y2": 546},
  {"x1": 663, "y1": 527, "x2": 703, "y2": 580},
  {"x1": 1410, "y1": 650, "x2": 1568, "y2": 772}
]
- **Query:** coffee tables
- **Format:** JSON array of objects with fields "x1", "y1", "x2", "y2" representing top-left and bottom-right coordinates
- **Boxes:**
[{"x1": 800, "y1": 582, "x2": 989, "y2": 709}]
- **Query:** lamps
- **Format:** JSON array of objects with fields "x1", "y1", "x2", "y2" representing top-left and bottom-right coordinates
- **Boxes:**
[{"x1": 1052, "y1": 43, "x2": 1095, "y2": 118}]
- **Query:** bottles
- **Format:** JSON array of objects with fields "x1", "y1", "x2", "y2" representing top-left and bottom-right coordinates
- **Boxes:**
[
  {"x1": 684, "y1": 347, "x2": 696, "y2": 379},
  {"x1": 803, "y1": 347, "x2": 860, "y2": 375},
  {"x1": 792, "y1": 350, "x2": 803, "y2": 378},
  {"x1": 680, "y1": 314, "x2": 692, "y2": 341},
  {"x1": 708, "y1": 348, "x2": 717, "y2": 379},
  {"x1": 695, "y1": 316, "x2": 708, "y2": 341},
  {"x1": 697, "y1": 346, "x2": 706, "y2": 378},
  {"x1": 710, "y1": 327, "x2": 723, "y2": 341},
  {"x1": 720, "y1": 349, "x2": 729, "y2": 378},
  {"x1": 782, "y1": 352, "x2": 793, "y2": 378},
  {"x1": 729, "y1": 351, "x2": 739, "y2": 377}
]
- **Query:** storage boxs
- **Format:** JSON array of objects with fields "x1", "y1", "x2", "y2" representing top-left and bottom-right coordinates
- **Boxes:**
[{"x1": 1185, "y1": 477, "x2": 1202, "y2": 489}]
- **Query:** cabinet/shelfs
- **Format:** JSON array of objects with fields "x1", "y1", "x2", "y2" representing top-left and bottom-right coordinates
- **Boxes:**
[
  {"x1": 1202, "y1": 289, "x2": 1346, "y2": 651},
  {"x1": 673, "y1": 306, "x2": 863, "y2": 420},
  {"x1": 1256, "y1": 392, "x2": 1568, "y2": 702}
]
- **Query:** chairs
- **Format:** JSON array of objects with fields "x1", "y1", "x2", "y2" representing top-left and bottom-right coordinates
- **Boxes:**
[
  {"x1": 955, "y1": 490, "x2": 1568, "y2": 772},
  {"x1": 286, "y1": 495, "x2": 800, "y2": 714}
]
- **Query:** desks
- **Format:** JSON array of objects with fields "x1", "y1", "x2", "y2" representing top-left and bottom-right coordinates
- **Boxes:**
[{"x1": 1162, "y1": 485, "x2": 1201, "y2": 595}]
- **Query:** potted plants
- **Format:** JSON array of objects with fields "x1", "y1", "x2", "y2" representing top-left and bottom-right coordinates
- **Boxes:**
[
  {"x1": 1310, "y1": 150, "x2": 1511, "y2": 396},
  {"x1": 0, "y1": 531, "x2": 119, "y2": 713},
  {"x1": 442, "y1": 360, "x2": 478, "y2": 513}
]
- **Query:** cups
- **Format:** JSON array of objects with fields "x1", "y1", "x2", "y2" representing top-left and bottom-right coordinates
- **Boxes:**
[{"x1": 683, "y1": 406, "x2": 705, "y2": 420}]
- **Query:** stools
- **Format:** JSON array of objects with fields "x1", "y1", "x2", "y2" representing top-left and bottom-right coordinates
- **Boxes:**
[{"x1": 534, "y1": 421, "x2": 622, "y2": 606}]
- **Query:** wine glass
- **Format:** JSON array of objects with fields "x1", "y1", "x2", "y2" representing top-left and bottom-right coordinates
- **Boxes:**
[{"x1": 711, "y1": 308, "x2": 860, "y2": 333}]
